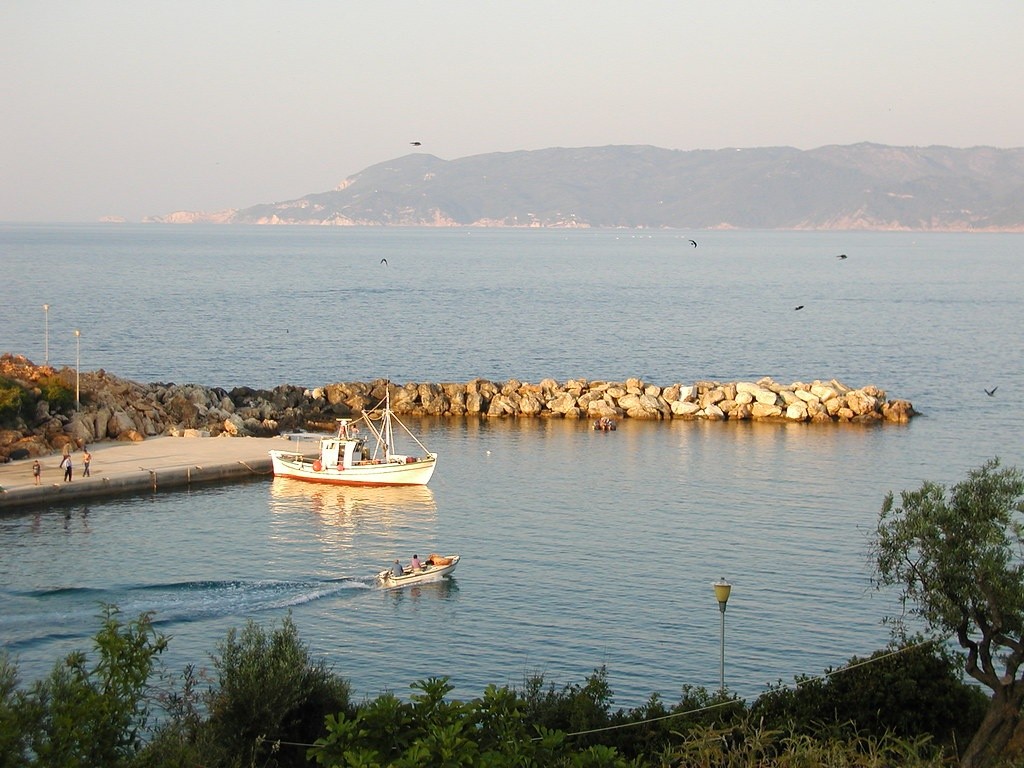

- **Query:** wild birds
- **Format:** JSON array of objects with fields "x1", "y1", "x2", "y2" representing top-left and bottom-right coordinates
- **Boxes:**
[
  {"x1": 381, "y1": 258, "x2": 387, "y2": 265},
  {"x1": 837, "y1": 254, "x2": 847, "y2": 260},
  {"x1": 984, "y1": 386, "x2": 998, "y2": 397},
  {"x1": 689, "y1": 239, "x2": 698, "y2": 248},
  {"x1": 411, "y1": 142, "x2": 421, "y2": 146},
  {"x1": 794, "y1": 305, "x2": 804, "y2": 310}
]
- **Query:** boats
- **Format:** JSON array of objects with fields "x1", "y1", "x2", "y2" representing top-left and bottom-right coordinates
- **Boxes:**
[
  {"x1": 376, "y1": 553, "x2": 462, "y2": 591},
  {"x1": 267, "y1": 371, "x2": 438, "y2": 486}
]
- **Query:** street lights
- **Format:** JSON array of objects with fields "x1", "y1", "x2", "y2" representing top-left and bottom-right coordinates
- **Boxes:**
[
  {"x1": 43, "y1": 304, "x2": 51, "y2": 366},
  {"x1": 74, "y1": 328, "x2": 80, "y2": 411},
  {"x1": 712, "y1": 576, "x2": 733, "y2": 697}
]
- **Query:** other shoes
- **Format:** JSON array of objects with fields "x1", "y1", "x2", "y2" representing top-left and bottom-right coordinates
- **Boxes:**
[
  {"x1": 88, "y1": 474, "x2": 90, "y2": 477},
  {"x1": 64, "y1": 478, "x2": 66, "y2": 481},
  {"x1": 69, "y1": 480, "x2": 72, "y2": 481},
  {"x1": 83, "y1": 474, "x2": 85, "y2": 477},
  {"x1": 60, "y1": 466, "x2": 62, "y2": 468}
]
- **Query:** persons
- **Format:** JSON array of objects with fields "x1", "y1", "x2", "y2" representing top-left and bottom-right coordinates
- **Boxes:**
[
  {"x1": 411, "y1": 554, "x2": 421, "y2": 571},
  {"x1": 350, "y1": 424, "x2": 360, "y2": 438},
  {"x1": 59, "y1": 442, "x2": 72, "y2": 482},
  {"x1": 393, "y1": 559, "x2": 404, "y2": 577},
  {"x1": 32, "y1": 460, "x2": 42, "y2": 485},
  {"x1": 81, "y1": 449, "x2": 92, "y2": 477}
]
